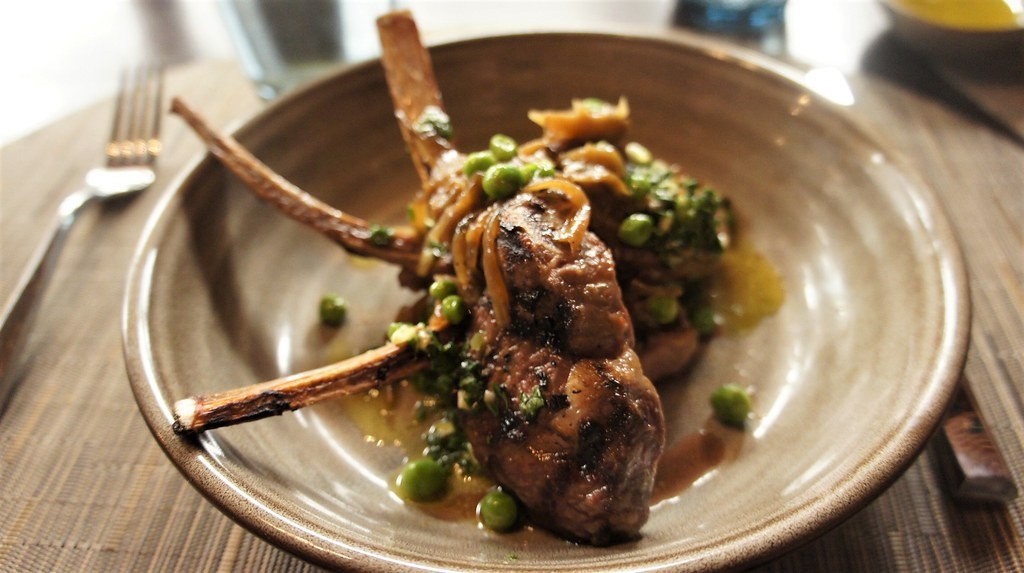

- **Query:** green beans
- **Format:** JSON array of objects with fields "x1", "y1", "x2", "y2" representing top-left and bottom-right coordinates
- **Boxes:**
[{"x1": 320, "y1": 134, "x2": 753, "y2": 532}]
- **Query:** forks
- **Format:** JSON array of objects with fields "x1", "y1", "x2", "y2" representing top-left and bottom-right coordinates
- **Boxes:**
[{"x1": 0, "y1": 60, "x2": 180, "y2": 408}]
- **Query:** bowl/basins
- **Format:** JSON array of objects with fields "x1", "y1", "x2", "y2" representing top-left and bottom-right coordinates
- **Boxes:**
[{"x1": 119, "y1": 29, "x2": 970, "y2": 573}]
